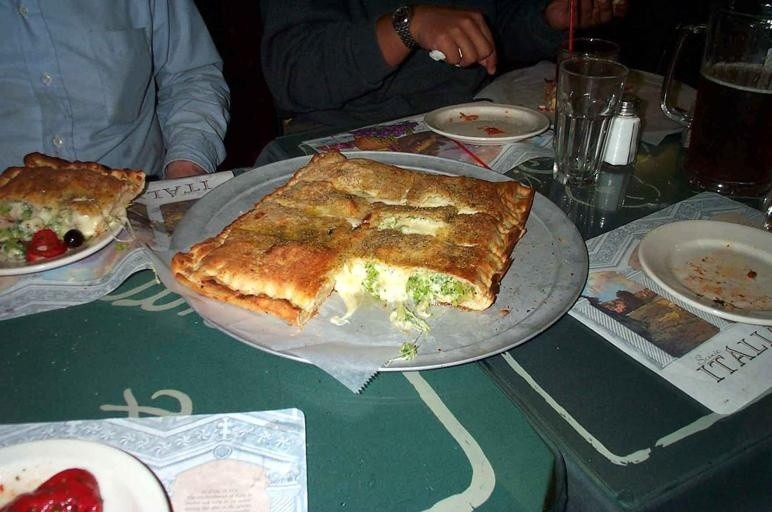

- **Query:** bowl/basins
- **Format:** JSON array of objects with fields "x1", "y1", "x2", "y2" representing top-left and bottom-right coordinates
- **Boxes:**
[{"x1": 692, "y1": 60, "x2": 772, "y2": 182}]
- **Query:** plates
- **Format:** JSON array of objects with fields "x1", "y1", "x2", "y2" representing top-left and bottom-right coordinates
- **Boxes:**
[
  {"x1": 1, "y1": 440, "x2": 171, "y2": 512},
  {"x1": 423, "y1": 102, "x2": 549, "y2": 145},
  {"x1": 639, "y1": 219, "x2": 772, "y2": 328},
  {"x1": 168, "y1": 151, "x2": 590, "y2": 372},
  {"x1": 0, "y1": 213, "x2": 127, "y2": 276}
]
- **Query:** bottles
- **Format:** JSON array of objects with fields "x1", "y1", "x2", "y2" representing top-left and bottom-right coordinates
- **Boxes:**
[{"x1": 602, "y1": 98, "x2": 638, "y2": 168}]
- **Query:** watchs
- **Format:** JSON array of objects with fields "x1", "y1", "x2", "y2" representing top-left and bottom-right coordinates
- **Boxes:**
[{"x1": 392, "y1": 3, "x2": 420, "y2": 50}]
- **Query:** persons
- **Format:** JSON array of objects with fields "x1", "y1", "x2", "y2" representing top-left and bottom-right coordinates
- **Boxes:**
[
  {"x1": 0, "y1": 0, "x2": 232, "y2": 180},
  {"x1": 247, "y1": 0, "x2": 628, "y2": 170}
]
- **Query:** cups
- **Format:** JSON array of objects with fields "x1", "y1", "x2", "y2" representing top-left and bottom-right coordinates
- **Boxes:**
[
  {"x1": 552, "y1": 58, "x2": 628, "y2": 185},
  {"x1": 557, "y1": 37, "x2": 622, "y2": 64},
  {"x1": 658, "y1": 16, "x2": 730, "y2": 129}
]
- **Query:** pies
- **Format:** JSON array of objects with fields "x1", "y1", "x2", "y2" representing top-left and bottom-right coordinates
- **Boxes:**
[
  {"x1": 169, "y1": 146, "x2": 536, "y2": 324},
  {"x1": 0, "y1": 150, "x2": 146, "y2": 237}
]
